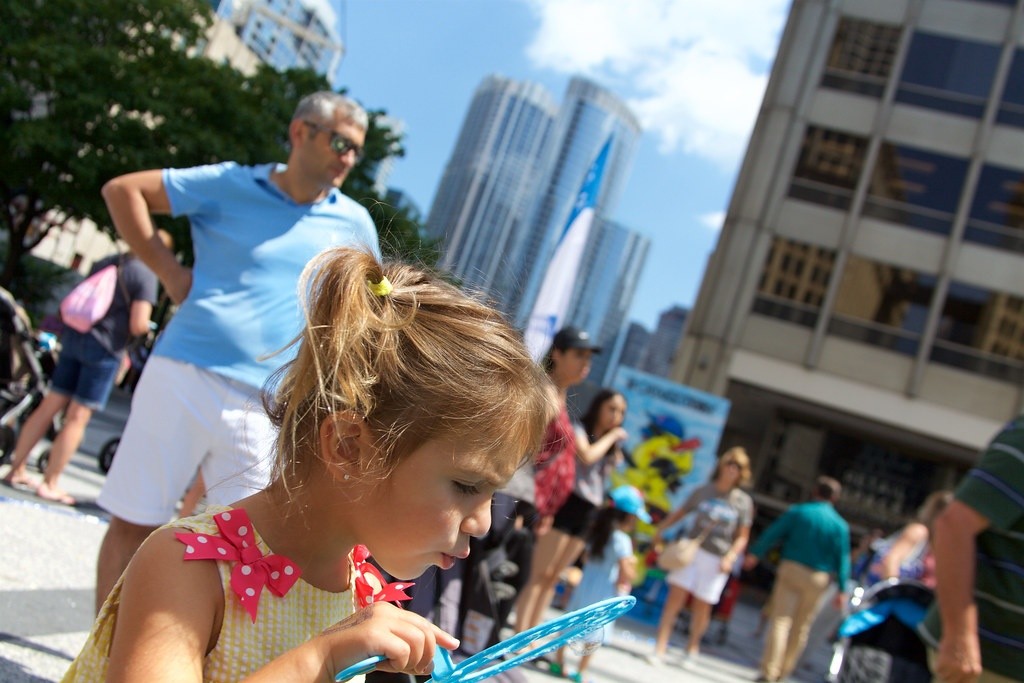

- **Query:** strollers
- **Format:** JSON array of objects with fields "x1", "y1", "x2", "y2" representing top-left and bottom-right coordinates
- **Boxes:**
[{"x1": 1, "y1": 311, "x2": 62, "y2": 475}]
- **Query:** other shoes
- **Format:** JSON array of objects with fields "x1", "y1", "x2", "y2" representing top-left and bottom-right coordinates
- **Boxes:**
[
  {"x1": 569, "y1": 670, "x2": 584, "y2": 683},
  {"x1": 549, "y1": 663, "x2": 569, "y2": 676}
]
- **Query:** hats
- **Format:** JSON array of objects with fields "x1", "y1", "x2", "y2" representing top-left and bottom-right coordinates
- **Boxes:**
[
  {"x1": 605, "y1": 483, "x2": 652, "y2": 522},
  {"x1": 554, "y1": 325, "x2": 603, "y2": 354}
]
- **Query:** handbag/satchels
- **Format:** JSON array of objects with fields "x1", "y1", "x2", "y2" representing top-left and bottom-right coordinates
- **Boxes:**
[{"x1": 658, "y1": 535, "x2": 702, "y2": 570}]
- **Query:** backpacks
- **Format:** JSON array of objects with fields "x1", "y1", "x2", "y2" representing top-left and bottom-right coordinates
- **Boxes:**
[{"x1": 60, "y1": 254, "x2": 138, "y2": 334}]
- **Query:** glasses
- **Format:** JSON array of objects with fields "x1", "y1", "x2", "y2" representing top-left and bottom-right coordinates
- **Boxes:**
[{"x1": 302, "y1": 121, "x2": 365, "y2": 163}]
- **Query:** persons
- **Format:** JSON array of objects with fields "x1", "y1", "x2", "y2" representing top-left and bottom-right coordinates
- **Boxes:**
[
  {"x1": 549, "y1": 483, "x2": 654, "y2": 683},
  {"x1": 650, "y1": 446, "x2": 756, "y2": 664},
  {"x1": 441, "y1": 325, "x2": 626, "y2": 658},
  {"x1": 505, "y1": 388, "x2": 628, "y2": 661},
  {"x1": 745, "y1": 476, "x2": 852, "y2": 683},
  {"x1": 93, "y1": 91, "x2": 377, "y2": 637},
  {"x1": 59, "y1": 245, "x2": 567, "y2": 683},
  {"x1": 1, "y1": 228, "x2": 174, "y2": 505},
  {"x1": 918, "y1": 412, "x2": 1024, "y2": 683},
  {"x1": 829, "y1": 490, "x2": 1024, "y2": 683}
]
